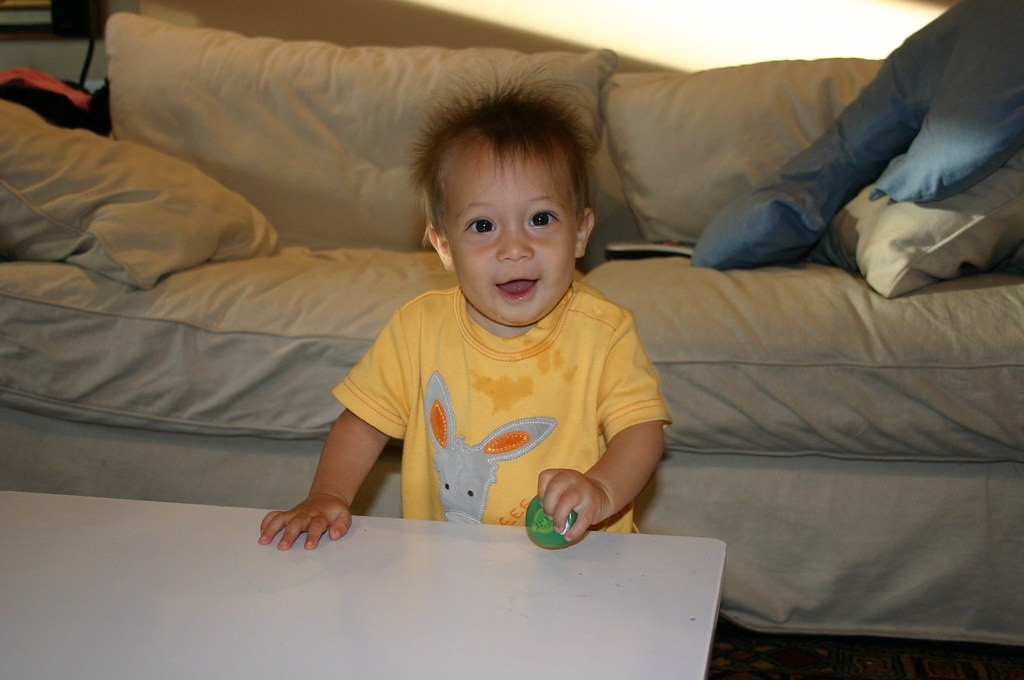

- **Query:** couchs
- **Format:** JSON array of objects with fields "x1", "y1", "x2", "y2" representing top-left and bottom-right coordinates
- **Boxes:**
[{"x1": 0, "y1": 12, "x2": 1024, "y2": 652}]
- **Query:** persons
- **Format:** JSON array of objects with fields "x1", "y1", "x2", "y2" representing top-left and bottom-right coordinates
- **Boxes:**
[{"x1": 258, "y1": 78, "x2": 673, "y2": 550}]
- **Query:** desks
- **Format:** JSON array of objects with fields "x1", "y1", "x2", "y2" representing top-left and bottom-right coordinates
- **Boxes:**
[{"x1": 0, "y1": 489, "x2": 729, "y2": 680}]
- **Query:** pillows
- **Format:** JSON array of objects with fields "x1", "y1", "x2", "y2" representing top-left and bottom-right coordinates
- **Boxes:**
[
  {"x1": 107, "y1": 10, "x2": 646, "y2": 288},
  {"x1": 0, "y1": 96, "x2": 287, "y2": 284},
  {"x1": 600, "y1": 55, "x2": 1024, "y2": 298}
]
